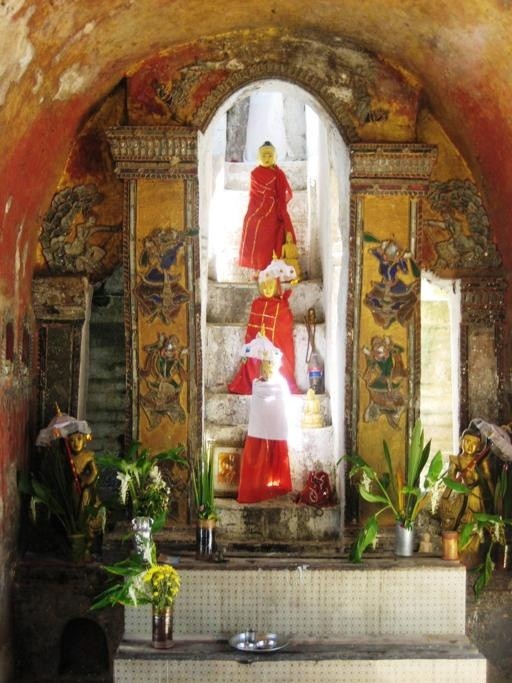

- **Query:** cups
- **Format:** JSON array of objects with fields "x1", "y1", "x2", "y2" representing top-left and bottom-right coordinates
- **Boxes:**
[{"x1": 245, "y1": 627, "x2": 256, "y2": 649}]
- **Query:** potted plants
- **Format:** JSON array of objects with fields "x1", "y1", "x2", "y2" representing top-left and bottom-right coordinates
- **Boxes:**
[
  {"x1": 170, "y1": 446, "x2": 222, "y2": 563},
  {"x1": 16, "y1": 432, "x2": 113, "y2": 566},
  {"x1": 344, "y1": 413, "x2": 494, "y2": 568}
]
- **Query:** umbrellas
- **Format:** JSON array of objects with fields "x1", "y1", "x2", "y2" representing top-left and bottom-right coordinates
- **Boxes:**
[
  {"x1": 441, "y1": 417, "x2": 512, "y2": 501},
  {"x1": 255, "y1": 251, "x2": 295, "y2": 296},
  {"x1": 35, "y1": 400, "x2": 88, "y2": 502}
]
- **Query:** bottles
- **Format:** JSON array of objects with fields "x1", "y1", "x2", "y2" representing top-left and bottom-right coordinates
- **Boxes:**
[{"x1": 307, "y1": 353, "x2": 324, "y2": 395}]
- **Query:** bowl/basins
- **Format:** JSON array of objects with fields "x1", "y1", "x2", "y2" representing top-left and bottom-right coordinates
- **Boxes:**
[{"x1": 229, "y1": 631, "x2": 289, "y2": 653}]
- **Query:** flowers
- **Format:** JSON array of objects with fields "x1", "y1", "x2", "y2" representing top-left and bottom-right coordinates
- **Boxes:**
[
  {"x1": 143, "y1": 564, "x2": 181, "y2": 611},
  {"x1": 95, "y1": 444, "x2": 187, "y2": 541}
]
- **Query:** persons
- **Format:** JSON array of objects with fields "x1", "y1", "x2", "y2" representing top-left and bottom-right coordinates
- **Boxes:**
[
  {"x1": 65, "y1": 211, "x2": 118, "y2": 265},
  {"x1": 235, "y1": 336, "x2": 292, "y2": 503},
  {"x1": 61, "y1": 431, "x2": 97, "y2": 531},
  {"x1": 440, "y1": 429, "x2": 491, "y2": 565},
  {"x1": 360, "y1": 335, "x2": 408, "y2": 428},
  {"x1": 139, "y1": 331, "x2": 186, "y2": 428},
  {"x1": 364, "y1": 239, "x2": 419, "y2": 328},
  {"x1": 133, "y1": 227, "x2": 191, "y2": 324},
  {"x1": 229, "y1": 265, "x2": 306, "y2": 394},
  {"x1": 423, "y1": 209, "x2": 472, "y2": 266},
  {"x1": 237, "y1": 141, "x2": 292, "y2": 281}
]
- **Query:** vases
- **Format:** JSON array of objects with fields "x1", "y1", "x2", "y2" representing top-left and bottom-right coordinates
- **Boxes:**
[
  {"x1": 152, "y1": 603, "x2": 174, "y2": 649},
  {"x1": 131, "y1": 515, "x2": 152, "y2": 564}
]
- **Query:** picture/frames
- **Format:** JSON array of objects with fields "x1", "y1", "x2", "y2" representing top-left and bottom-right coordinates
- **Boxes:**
[{"x1": 206, "y1": 440, "x2": 245, "y2": 498}]
